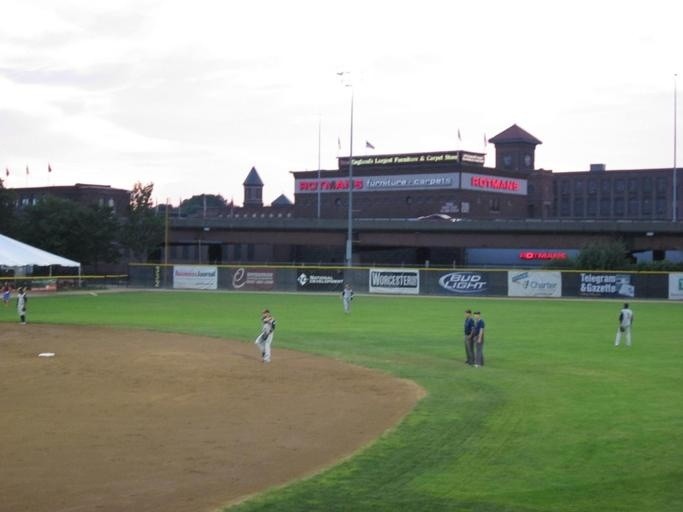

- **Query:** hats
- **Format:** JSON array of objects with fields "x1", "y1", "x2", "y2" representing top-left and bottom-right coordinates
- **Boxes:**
[
  {"x1": 464, "y1": 309, "x2": 481, "y2": 315},
  {"x1": 262, "y1": 308, "x2": 270, "y2": 314}
]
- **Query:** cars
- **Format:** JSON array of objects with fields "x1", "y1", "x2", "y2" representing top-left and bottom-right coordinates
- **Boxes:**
[{"x1": 418, "y1": 214, "x2": 463, "y2": 223}]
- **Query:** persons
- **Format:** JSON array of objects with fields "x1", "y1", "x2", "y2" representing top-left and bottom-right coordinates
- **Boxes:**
[
  {"x1": 463, "y1": 309, "x2": 476, "y2": 365},
  {"x1": 15, "y1": 286, "x2": 27, "y2": 324},
  {"x1": 340, "y1": 282, "x2": 355, "y2": 313},
  {"x1": 472, "y1": 311, "x2": 484, "y2": 368},
  {"x1": 614, "y1": 303, "x2": 633, "y2": 348},
  {"x1": 0, "y1": 281, "x2": 12, "y2": 305},
  {"x1": 254, "y1": 308, "x2": 277, "y2": 363}
]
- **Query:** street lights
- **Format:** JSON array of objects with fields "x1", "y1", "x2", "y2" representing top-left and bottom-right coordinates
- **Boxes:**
[
  {"x1": 317, "y1": 71, "x2": 350, "y2": 218},
  {"x1": 672, "y1": 72, "x2": 679, "y2": 223},
  {"x1": 345, "y1": 83, "x2": 354, "y2": 266}
]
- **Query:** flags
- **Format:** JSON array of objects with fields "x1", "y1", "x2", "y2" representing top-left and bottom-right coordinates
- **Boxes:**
[{"x1": 365, "y1": 141, "x2": 374, "y2": 150}]
